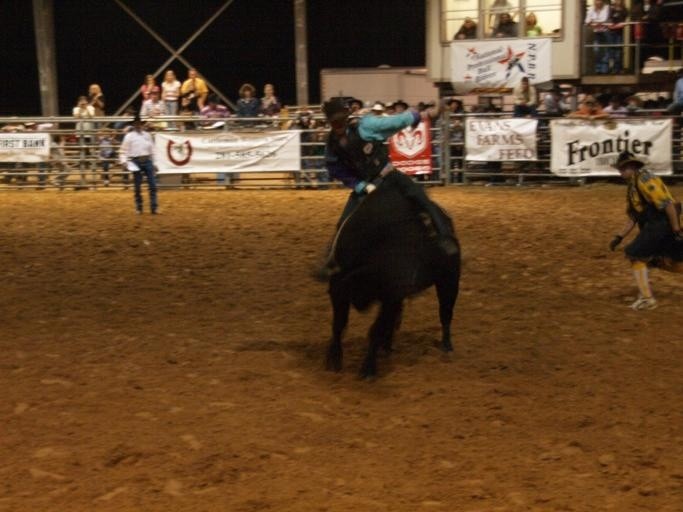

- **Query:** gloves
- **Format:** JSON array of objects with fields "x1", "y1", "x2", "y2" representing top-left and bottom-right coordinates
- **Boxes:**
[{"x1": 610, "y1": 235, "x2": 622, "y2": 252}]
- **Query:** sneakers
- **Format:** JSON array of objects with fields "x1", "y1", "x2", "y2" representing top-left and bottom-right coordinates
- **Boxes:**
[
  {"x1": 625, "y1": 297, "x2": 657, "y2": 311},
  {"x1": 311, "y1": 261, "x2": 341, "y2": 276}
]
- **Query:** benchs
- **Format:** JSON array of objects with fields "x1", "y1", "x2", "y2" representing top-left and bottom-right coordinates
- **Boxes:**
[{"x1": 1, "y1": 2, "x2": 682, "y2": 177}]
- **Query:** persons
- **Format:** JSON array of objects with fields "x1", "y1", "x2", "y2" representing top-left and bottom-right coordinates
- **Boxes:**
[
  {"x1": 446, "y1": 0, "x2": 683, "y2": 185},
  {"x1": 117, "y1": 117, "x2": 162, "y2": 215},
  {"x1": 312, "y1": 94, "x2": 455, "y2": 281},
  {"x1": 607, "y1": 149, "x2": 682, "y2": 312},
  {"x1": 0, "y1": 67, "x2": 445, "y2": 189}
]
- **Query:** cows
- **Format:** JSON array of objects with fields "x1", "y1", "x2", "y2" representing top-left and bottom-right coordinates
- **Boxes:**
[{"x1": 324, "y1": 168, "x2": 461, "y2": 382}]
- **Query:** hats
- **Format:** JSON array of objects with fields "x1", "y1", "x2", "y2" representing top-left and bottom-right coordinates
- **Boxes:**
[
  {"x1": 369, "y1": 103, "x2": 385, "y2": 111},
  {"x1": 610, "y1": 154, "x2": 644, "y2": 169},
  {"x1": 323, "y1": 97, "x2": 353, "y2": 120},
  {"x1": 239, "y1": 83, "x2": 256, "y2": 96},
  {"x1": 147, "y1": 85, "x2": 161, "y2": 95},
  {"x1": 392, "y1": 100, "x2": 408, "y2": 110},
  {"x1": 129, "y1": 117, "x2": 147, "y2": 127},
  {"x1": 348, "y1": 100, "x2": 363, "y2": 109},
  {"x1": 415, "y1": 102, "x2": 429, "y2": 110}
]
